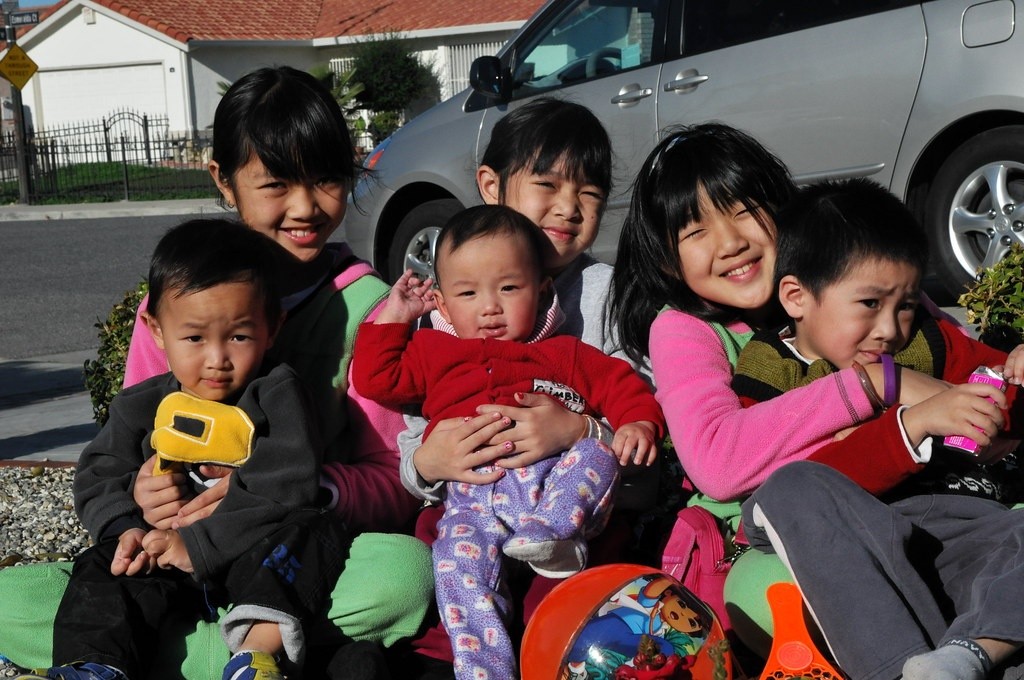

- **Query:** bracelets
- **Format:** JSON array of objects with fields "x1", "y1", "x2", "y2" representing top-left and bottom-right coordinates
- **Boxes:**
[
  {"x1": 851, "y1": 360, "x2": 884, "y2": 411},
  {"x1": 877, "y1": 354, "x2": 896, "y2": 404}
]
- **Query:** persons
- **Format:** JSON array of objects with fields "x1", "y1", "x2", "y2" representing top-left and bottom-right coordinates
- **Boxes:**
[
  {"x1": 352, "y1": 205, "x2": 666, "y2": 680},
  {"x1": 13, "y1": 219, "x2": 349, "y2": 680},
  {"x1": 734, "y1": 177, "x2": 1024, "y2": 680},
  {"x1": 394, "y1": 97, "x2": 656, "y2": 663},
  {"x1": 0, "y1": 64, "x2": 435, "y2": 680},
  {"x1": 613, "y1": 122, "x2": 1024, "y2": 661}
]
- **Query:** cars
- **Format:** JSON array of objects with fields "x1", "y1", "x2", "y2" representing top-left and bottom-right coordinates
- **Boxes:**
[{"x1": 346, "y1": 0, "x2": 1023, "y2": 305}]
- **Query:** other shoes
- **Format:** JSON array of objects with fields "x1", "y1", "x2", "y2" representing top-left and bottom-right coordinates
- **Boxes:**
[
  {"x1": 222, "y1": 652, "x2": 285, "y2": 680},
  {"x1": 13, "y1": 662, "x2": 128, "y2": 680}
]
